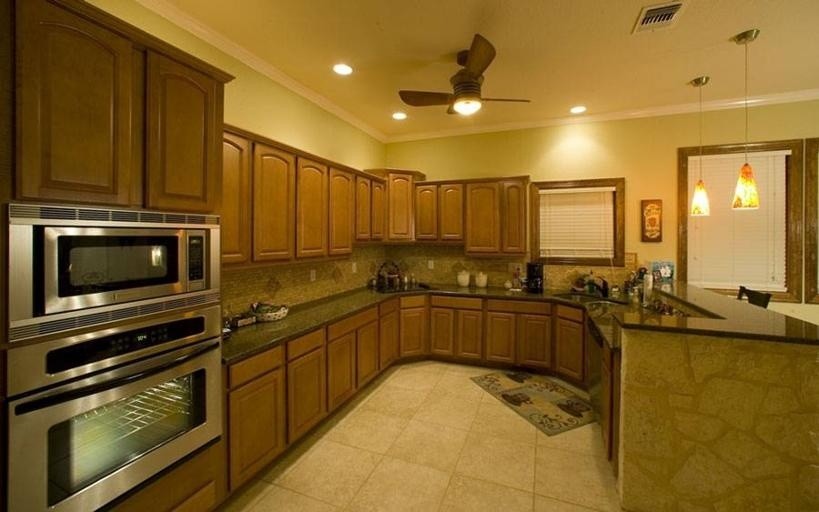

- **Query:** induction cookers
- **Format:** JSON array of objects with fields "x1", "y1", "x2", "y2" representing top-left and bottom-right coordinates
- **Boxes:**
[{"x1": 364, "y1": 281, "x2": 438, "y2": 295}]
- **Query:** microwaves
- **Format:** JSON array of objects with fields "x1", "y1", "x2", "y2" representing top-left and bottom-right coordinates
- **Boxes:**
[{"x1": 4, "y1": 201, "x2": 224, "y2": 351}]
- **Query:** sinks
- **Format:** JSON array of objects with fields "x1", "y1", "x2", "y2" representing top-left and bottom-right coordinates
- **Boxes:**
[
  {"x1": 583, "y1": 298, "x2": 629, "y2": 309},
  {"x1": 553, "y1": 293, "x2": 602, "y2": 302}
]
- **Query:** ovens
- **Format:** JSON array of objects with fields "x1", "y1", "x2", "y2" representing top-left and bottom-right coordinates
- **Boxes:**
[{"x1": 2, "y1": 305, "x2": 224, "y2": 510}]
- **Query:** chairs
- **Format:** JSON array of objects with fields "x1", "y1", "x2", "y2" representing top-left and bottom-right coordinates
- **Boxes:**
[{"x1": 737, "y1": 285, "x2": 773, "y2": 308}]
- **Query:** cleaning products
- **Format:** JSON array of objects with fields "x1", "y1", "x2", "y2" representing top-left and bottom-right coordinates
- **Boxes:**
[{"x1": 589, "y1": 271, "x2": 595, "y2": 293}]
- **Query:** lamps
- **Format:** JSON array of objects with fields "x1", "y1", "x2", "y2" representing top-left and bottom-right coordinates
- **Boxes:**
[
  {"x1": 452, "y1": 88, "x2": 482, "y2": 118},
  {"x1": 731, "y1": 28, "x2": 760, "y2": 212},
  {"x1": 691, "y1": 74, "x2": 711, "y2": 217}
]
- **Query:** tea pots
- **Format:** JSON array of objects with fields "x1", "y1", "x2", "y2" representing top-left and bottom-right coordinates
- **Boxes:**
[{"x1": 371, "y1": 258, "x2": 402, "y2": 295}]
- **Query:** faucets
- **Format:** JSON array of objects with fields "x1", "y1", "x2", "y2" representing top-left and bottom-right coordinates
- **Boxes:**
[{"x1": 584, "y1": 276, "x2": 608, "y2": 298}]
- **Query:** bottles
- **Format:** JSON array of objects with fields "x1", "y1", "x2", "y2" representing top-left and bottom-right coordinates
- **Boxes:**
[
  {"x1": 402, "y1": 283, "x2": 416, "y2": 291},
  {"x1": 401, "y1": 271, "x2": 417, "y2": 283},
  {"x1": 585, "y1": 264, "x2": 656, "y2": 302}
]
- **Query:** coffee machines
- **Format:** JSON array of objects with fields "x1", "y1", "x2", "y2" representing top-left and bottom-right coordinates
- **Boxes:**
[{"x1": 526, "y1": 261, "x2": 547, "y2": 293}]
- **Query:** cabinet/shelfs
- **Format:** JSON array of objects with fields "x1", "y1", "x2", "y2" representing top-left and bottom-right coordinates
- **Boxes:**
[
  {"x1": 95, "y1": 439, "x2": 223, "y2": 512},
  {"x1": 0, "y1": 1, "x2": 236, "y2": 217},
  {"x1": 227, "y1": 292, "x2": 622, "y2": 503},
  {"x1": 219, "y1": 122, "x2": 530, "y2": 271}
]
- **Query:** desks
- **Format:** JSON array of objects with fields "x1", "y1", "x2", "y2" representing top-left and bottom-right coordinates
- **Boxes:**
[{"x1": 610, "y1": 276, "x2": 818, "y2": 512}]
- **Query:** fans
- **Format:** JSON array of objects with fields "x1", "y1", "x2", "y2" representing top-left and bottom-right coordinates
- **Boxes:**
[{"x1": 397, "y1": 31, "x2": 533, "y2": 114}]
